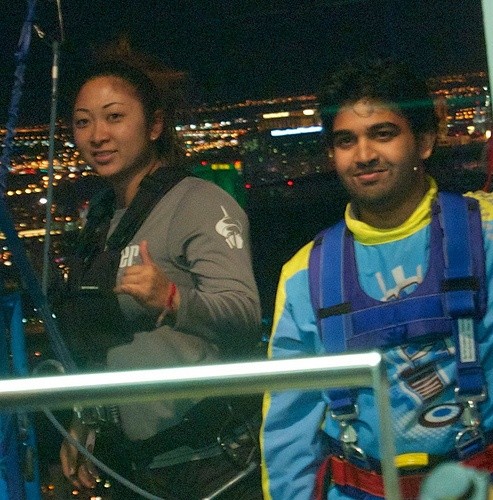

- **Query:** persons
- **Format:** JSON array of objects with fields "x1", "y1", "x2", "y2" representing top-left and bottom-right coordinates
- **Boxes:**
[
  {"x1": 260, "y1": 57, "x2": 493, "y2": 500},
  {"x1": 59, "y1": 64, "x2": 263, "y2": 500}
]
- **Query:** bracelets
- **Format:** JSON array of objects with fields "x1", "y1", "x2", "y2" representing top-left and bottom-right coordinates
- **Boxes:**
[{"x1": 163, "y1": 282, "x2": 176, "y2": 318}]
